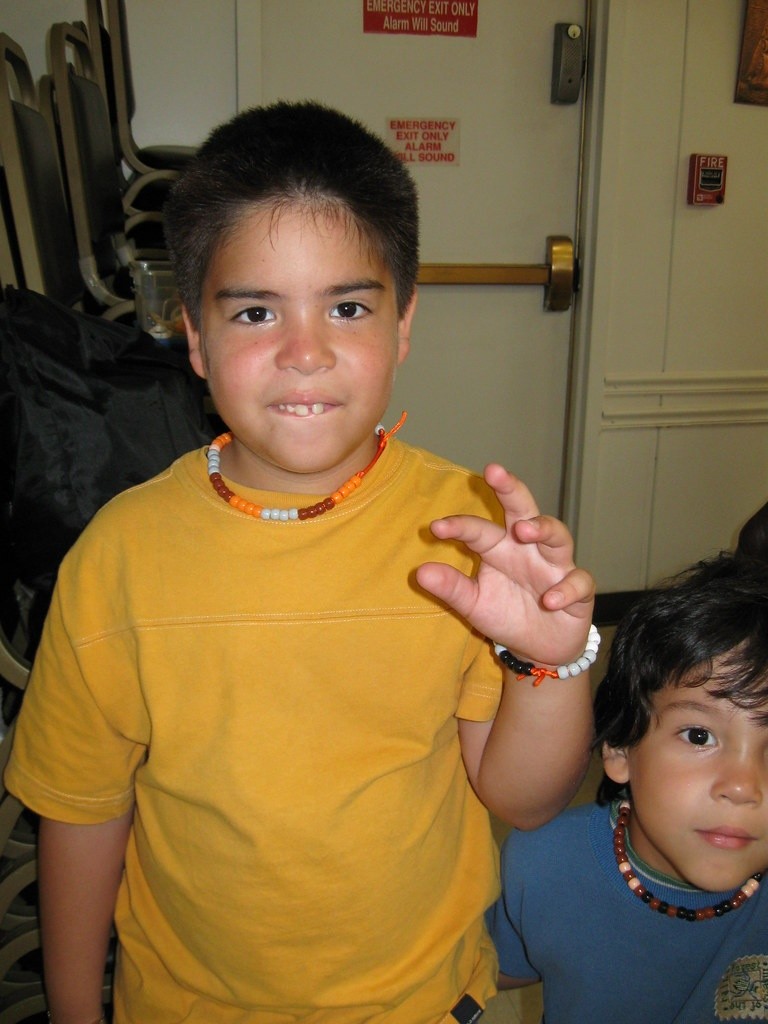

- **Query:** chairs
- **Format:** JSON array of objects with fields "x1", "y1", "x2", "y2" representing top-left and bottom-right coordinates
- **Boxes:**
[{"x1": 0, "y1": 0, "x2": 201, "y2": 329}]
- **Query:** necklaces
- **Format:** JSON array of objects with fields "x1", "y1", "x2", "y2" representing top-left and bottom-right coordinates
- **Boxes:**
[
  {"x1": 613, "y1": 800, "x2": 762, "y2": 921},
  {"x1": 208, "y1": 422, "x2": 384, "y2": 521}
]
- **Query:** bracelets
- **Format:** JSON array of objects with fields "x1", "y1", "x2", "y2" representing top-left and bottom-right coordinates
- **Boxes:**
[{"x1": 490, "y1": 624, "x2": 600, "y2": 686}]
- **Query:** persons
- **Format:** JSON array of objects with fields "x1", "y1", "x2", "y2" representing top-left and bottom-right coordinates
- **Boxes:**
[
  {"x1": 486, "y1": 551, "x2": 767, "y2": 1023},
  {"x1": 0, "y1": 105, "x2": 595, "y2": 1024}
]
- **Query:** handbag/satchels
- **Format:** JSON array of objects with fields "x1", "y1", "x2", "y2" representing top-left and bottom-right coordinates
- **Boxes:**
[{"x1": 0, "y1": 285, "x2": 230, "y2": 596}]
